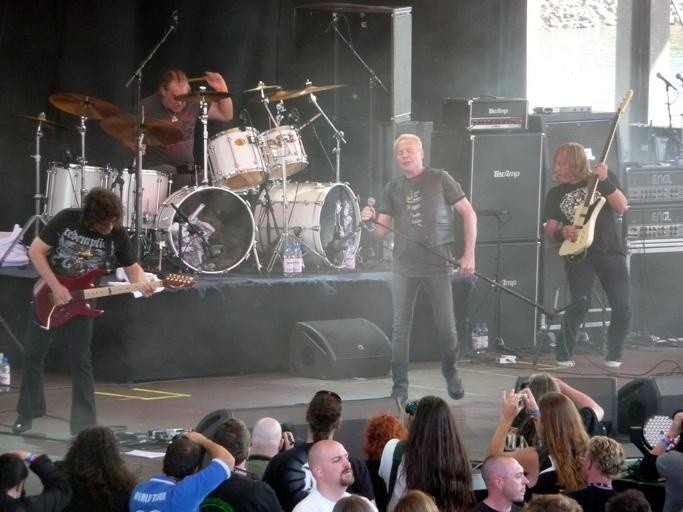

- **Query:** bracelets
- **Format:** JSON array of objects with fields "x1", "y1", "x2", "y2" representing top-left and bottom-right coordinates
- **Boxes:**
[
  {"x1": 25, "y1": 450, "x2": 35, "y2": 462},
  {"x1": 659, "y1": 434, "x2": 675, "y2": 447},
  {"x1": 525, "y1": 408, "x2": 540, "y2": 416}
]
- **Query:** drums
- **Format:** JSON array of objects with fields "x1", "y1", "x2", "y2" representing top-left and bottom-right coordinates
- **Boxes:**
[
  {"x1": 44, "y1": 161, "x2": 115, "y2": 221},
  {"x1": 260, "y1": 125, "x2": 309, "y2": 181},
  {"x1": 207, "y1": 127, "x2": 269, "y2": 190},
  {"x1": 112, "y1": 169, "x2": 172, "y2": 230},
  {"x1": 254, "y1": 181, "x2": 361, "y2": 269},
  {"x1": 154, "y1": 185, "x2": 256, "y2": 274}
]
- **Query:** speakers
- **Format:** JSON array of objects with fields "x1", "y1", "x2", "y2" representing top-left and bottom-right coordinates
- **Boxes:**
[
  {"x1": 291, "y1": 317, "x2": 394, "y2": 379},
  {"x1": 616, "y1": 371, "x2": 683, "y2": 435},
  {"x1": 514, "y1": 376, "x2": 617, "y2": 441}
]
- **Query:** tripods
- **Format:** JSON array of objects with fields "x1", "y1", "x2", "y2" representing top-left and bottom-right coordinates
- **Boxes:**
[
  {"x1": 371, "y1": 220, "x2": 586, "y2": 365},
  {"x1": 0, "y1": 111, "x2": 81, "y2": 266},
  {"x1": 465, "y1": 209, "x2": 527, "y2": 361},
  {"x1": 263, "y1": 99, "x2": 336, "y2": 273},
  {"x1": 125, "y1": 22, "x2": 189, "y2": 275}
]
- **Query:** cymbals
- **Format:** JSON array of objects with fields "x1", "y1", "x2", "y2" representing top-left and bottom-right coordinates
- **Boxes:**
[
  {"x1": 49, "y1": 92, "x2": 119, "y2": 119},
  {"x1": 280, "y1": 84, "x2": 348, "y2": 99},
  {"x1": 18, "y1": 111, "x2": 73, "y2": 135},
  {"x1": 174, "y1": 91, "x2": 234, "y2": 103},
  {"x1": 101, "y1": 114, "x2": 182, "y2": 146},
  {"x1": 250, "y1": 90, "x2": 298, "y2": 102},
  {"x1": 245, "y1": 84, "x2": 279, "y2": 93}
]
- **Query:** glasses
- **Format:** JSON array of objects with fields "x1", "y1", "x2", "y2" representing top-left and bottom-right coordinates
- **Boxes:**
[{"x1": 314, "y1": 389, "x2": 343, "y2": 406}]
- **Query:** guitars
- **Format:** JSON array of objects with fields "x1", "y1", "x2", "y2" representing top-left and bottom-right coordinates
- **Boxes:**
[
  {"x1": 558, "y1": 90, "x2": 633, "y2": 257},
  {"x1": 32, "y1": 269, "x2": 196, "y2": 328}
]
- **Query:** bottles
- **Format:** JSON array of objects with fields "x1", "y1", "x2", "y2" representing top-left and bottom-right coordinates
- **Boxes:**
[
  {"x1": 291, "y1": 244, "x2": 298, "y2": 276},
  {"x1": 345, "y1": 238, "x2": 356, "y2": 271},
  {"x1": 471, "y1": 323, "x2": 482, "y2": 350},
  {"x1": 296, "y1": 242, "x2": 304, "y2": 274},
  {"x1": 481, "y1": 323, "x2": 489, "y2": 349},
  {"x1": 0, "y1": 356, "x2": 10, "y2": 393},
  {"x1": 284, "y1": 244, "x2": 292, "y2": 276}
]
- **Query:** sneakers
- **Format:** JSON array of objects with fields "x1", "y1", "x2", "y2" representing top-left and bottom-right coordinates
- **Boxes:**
[
  {"x1": 604, "y1": 349, "x2": 621, "y2": 370},
  {"x1": 553, "y1": 358, "x2": 576, "y2": 368}
]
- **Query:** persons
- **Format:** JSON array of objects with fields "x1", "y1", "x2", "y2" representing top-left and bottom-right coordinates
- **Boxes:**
[
  {"x1": 379, "y1": 395, "x2": 475, "y2": 512},
  {"x1": 488, "y1": 385, "x2": 589, "y2": 500},
  {"x1": 542, "y1": 142, "x2": 631, "y2": 369},
  {"x1": 292, "y1": 441, "x2": 378, "y2": 512},
  {"x1": 129, "y1": 430, "x2": 235, "y2": 512},
  {"x1": 54, "y1": 426, "x2": 131, "y2": 512},
  {"x1": 262, "y1": 390, "x2": 377, "y2": 512},
  {"x1": 507, "y1": 371, "x2": 605, "y2": 445},
  {"x1": 362, "y1": 413, "x2": 406, "y2": 511},
  {"x1": 360, "y1": 133, "x2": 478, "y2": 406},
  {"x1": 651, "y1": 411, "x2": 683, "y2": 512},
  {"x1": 199, "y1": 419, "x2": 283, "y2": 512},
  {"x1": 467, "y1": 454, "x2": 528, "y2": 512},
  {"x1": 566, "y1": 435, "x2": 624, "y2": 512},
  {"x1": 243, "y1": 417, "x2": 295, "y2": 481},
  {"x1": 120, "y1": 65, "x2": 234, "y2": 192},
  {"x1": 0, "y1": 448, "x2": 72, "y2": 512},
  {"x1": 11, "y1": 187, "x2": 156, "y2": 435}
]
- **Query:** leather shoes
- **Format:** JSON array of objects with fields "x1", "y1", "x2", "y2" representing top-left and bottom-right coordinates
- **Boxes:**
[
  {"x1": 12, "y1": 414, "x2": 34, "y2": 434},
  {"x1": 390, "y1": 389, "x2": 409, "y2": 409},
  {"x1": 443, "y1": 368, "x2": 465, "y2": 399}
]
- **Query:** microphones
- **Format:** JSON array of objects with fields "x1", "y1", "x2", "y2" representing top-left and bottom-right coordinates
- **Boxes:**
[
  {"x1": 365, "y1": 194, "x2": 376, "y2": 224},
  {"x1": 675, "y1": 71, "x2": 683, "y2": 86},
  {"x1": 656, "y1": 72, "x2": 677, "y2": 90}
]
